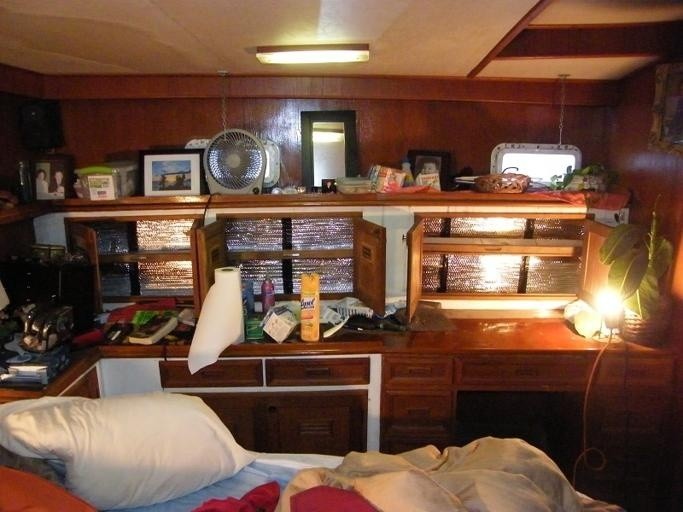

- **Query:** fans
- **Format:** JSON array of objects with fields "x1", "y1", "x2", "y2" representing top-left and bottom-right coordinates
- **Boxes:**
[{"x1": 203, "y1": 129, "x2": 267, "y2": 194}]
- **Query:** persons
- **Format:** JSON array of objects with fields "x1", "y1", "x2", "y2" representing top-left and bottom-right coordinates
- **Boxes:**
[
  {"x1": 36, "y1": 170, "x2": 48, "y2": 195},
  {"x1": 415, "y1": 158, "x2": 439, "y2": 187},
  {"x1": 323, "y1": 181, "x2": 334, "y2": 193},
  {"x1": 49, "y1": 171, "x2": 64, "y2": 195}
]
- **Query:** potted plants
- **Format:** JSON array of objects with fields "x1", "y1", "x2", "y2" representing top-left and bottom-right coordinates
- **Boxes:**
[{"x1": 600, "y1": 191, "x2": 676, "y2": 343}]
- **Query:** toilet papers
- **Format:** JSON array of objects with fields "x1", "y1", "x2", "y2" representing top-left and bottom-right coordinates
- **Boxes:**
[{"x1": 187, "y1": 267, "x2": 244, "y2": 375}]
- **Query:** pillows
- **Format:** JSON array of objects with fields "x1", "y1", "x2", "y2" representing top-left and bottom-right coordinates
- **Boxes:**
[{"x1": 2, "y1": 391, "x2": 255, "y2": 512}]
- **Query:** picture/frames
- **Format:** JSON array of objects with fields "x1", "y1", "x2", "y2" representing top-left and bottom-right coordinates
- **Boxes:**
[
  {"x1": 138, "y1": 149, "x2": 204, "y2": 196},
  {"x1": 32, "y1": 156, "x2": 70, "y2": 203},
  {"x1": 407, "y1": 148, "x2": 452, "y2": 193},
  {"x1": 646, "y1": 61, "x2": 682, "y2": 157}
]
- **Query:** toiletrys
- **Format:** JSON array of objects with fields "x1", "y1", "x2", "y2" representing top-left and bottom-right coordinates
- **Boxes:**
[{"x1": 242, "y1": 280, "x2": 254, "y2": 315}]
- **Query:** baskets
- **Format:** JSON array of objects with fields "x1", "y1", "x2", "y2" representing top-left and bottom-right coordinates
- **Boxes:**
[{"x1": 475, "y1": 173, "x2": 530, "y2": 193}]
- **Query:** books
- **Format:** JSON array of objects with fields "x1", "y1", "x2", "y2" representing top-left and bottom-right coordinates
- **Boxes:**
[{"x1": 103, "y1": 296, "x2": 196, "y2": 345}]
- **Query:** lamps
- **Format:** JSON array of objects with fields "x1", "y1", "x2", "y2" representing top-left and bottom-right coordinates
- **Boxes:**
[{"x1": 256, "y1": 43, "x2": 369, "y2": 65}]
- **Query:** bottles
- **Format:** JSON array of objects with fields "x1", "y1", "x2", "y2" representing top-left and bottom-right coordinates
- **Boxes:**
[
  {"x1": 261, "y1": 278, "x2": 276, "y2": 313},
  {"x1": 400, "y1": 162, "x2": 414, "y2": 186},
  {"x1": 15, "y1": 160, "x2": 32, "y2": 204}
]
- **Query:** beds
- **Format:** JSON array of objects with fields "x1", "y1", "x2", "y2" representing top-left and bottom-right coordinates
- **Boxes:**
[{"x1": 1, "y1": 443, "x2": 626, "y2": 511}]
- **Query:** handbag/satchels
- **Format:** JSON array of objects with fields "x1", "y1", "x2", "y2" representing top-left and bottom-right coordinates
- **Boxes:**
[{"x1": 21, "y1": 302, "x2": 76, "y2": 354}]
